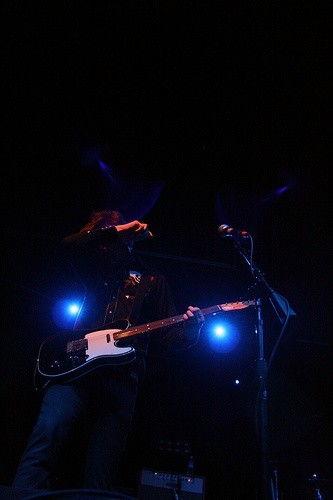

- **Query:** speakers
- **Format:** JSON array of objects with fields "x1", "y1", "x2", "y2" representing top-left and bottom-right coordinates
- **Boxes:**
[{"x1": 136, "y1": 468, "x2": 206, "y2": 500}]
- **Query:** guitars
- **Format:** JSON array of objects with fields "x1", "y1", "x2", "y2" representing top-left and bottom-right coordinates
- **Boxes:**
[{"x1": 35, "y1": 299, "x2": 258, "y2": 382}]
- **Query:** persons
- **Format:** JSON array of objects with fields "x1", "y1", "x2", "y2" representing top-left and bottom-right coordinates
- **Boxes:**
[{"x1": 10, "y1": 212, "x2": 205, "y2": 492}]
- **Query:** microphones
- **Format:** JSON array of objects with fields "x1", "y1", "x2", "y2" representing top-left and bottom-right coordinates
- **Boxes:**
[
  {"x1": 176, "y1": 477, "x2": 181, "y2": 493},
  {"x1": 217, "y1": 224, "x2": 248, "y2": 238}
]
